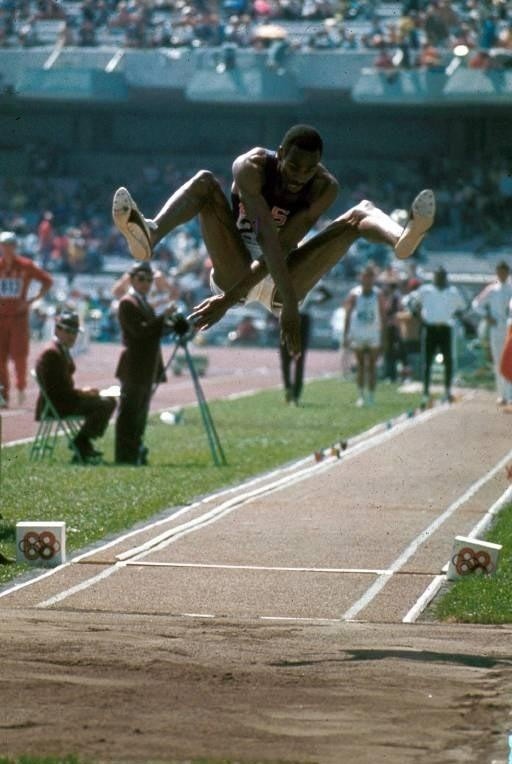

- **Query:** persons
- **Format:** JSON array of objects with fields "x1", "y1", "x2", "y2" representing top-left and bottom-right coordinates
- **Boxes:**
[
  {"x1": 114, "y1": 260, "x2": 178, "y2": 467},
  {"x1": 112, "y1": 126, "x2": 434, "y2": 366},
  {"x1": 34, "y1": 311, "x2": 116, "y2": 464}
]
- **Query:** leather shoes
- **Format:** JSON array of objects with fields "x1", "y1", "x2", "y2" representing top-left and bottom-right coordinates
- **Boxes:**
[{"x1": 67, "y1": 437, "x2": 106, "y2": 457}]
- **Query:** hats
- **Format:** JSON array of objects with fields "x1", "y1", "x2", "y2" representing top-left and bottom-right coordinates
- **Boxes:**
[
  {"x1": 54, "y1": 310, "x2": 86, "y2": 334},
  {"x1": 128, "y1": 261, "x2": 154, "y2": 274}
]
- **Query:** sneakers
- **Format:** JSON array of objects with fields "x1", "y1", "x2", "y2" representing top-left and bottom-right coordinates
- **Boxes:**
[
  {"x1": 283, "y1": 374, "x2": 512, "y2": 409},
  {"x1": 110, "y1": 186, "x2": 161, "y2": 263},
  {"x1": 391, "y1": 187, "x2": 437, "y2": 260}
]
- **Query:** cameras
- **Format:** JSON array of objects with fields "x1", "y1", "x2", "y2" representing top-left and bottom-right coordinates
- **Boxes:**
[{"x1": 174, "y1": 312, "x2": 192, "y2": 336}]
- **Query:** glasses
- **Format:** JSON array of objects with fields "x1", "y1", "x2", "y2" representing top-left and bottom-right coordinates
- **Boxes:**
[{"x1": 132, "y1": 278, "x2": 153, "y2": 282}]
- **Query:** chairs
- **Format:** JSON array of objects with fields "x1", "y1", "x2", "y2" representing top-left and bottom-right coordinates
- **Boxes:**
[{"x1": 30, "y1": 372, "x2": 87, "y2": 467}]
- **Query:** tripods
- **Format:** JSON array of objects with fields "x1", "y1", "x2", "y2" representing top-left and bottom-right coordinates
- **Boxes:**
[{"x1": 149, "y1": 336, "x2": 227, "y2": 469}]
